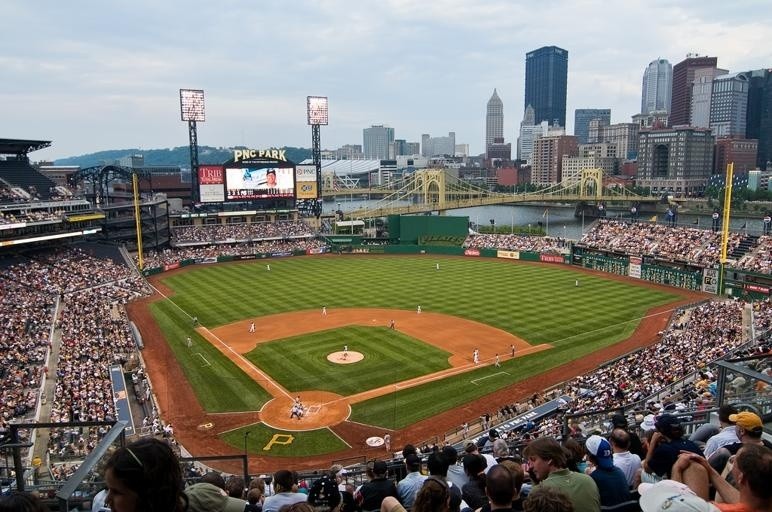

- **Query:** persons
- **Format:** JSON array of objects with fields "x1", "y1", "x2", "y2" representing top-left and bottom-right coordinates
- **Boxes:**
[
  {"x1": 344, "y1": 344, "x2": 348, "y2": 356},
  {"x1": 188, "y1": 336, "x2": 192, "y2": 347},
  {"x1": 1, "y1": 389, "x2": 771, "y2": 511},
  {"x1": 437, "y1": 263, "x2": 440, "y2": 270},
  {"x1": 193, "y1": 316, "x2": 198, "y2": 327},
  {"x1": 462, "y1": 234, "x2": 572, "y2": 256},
  {"x1": 249, "y1": 322, "x2": 255, "y2": 333},
  {"x1": 255, "y1": 169, "x2": 279, "y2": 189},
  {"x1": 391, "y1": 320, "x2": 395, "y2": 330},
  {"x1": 337, "y1": 239, "x2": 389, "y2": 256},
  {"x1": 579, "y1": 189, "x2": 772, "y2": 275},
  {"x1": 576, "y1": 279, "x2": 579, "y2": 287},
  {"x1": 418, "y1": 304, "x2": 422, "y2": 312},
  {"x1": 473, "y1": 343, "x2": 515, "y2": 368},
  {"x1": 565, "y1": 297, "x2": 771, "y2": 438},
  {"x1": 322, "y1": 305, "x2": 327, "y2": 315},
  {"x1": 164, "y1": 183, "x2": 331, "y2": 271},
  {"x1": 1, "y1": 184, "x2": 165, "y2": 459}
]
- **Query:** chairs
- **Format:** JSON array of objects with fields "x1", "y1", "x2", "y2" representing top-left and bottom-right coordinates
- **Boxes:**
[{"x1": 598, "y1": 488, "x2": 640, "y2": 511}]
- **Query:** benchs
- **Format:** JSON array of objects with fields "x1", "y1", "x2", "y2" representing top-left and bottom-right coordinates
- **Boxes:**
[
  {"x1": 725, "y1": 235, "x2": 760, "y2": 260},
  {"x1": 0, "y1": 160, "x2": 95, "y2": 225}
]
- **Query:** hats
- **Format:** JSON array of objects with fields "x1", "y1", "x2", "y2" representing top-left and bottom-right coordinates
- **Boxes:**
[
  {"x1": 586, "y1": 435, "x2": 613, "y2": 468},
  {"x1": 462, "y1": 439, "x2": 474, "y2": 449},
  {"x1": 720, "y1": 405, "x2": 764, "y2": 432},
  {"x1": 635, "y1": 399, "x2": 684, "y2": 431},
  {"x1": 185, "y1": 482, "x2": 246, "y2": 512},
  {"x1": 406, "y1": 455, "x2": 418, "y2": 464},
  {"x1": 373, "y1": 461, "x2": 386, "y2": 472}
]
